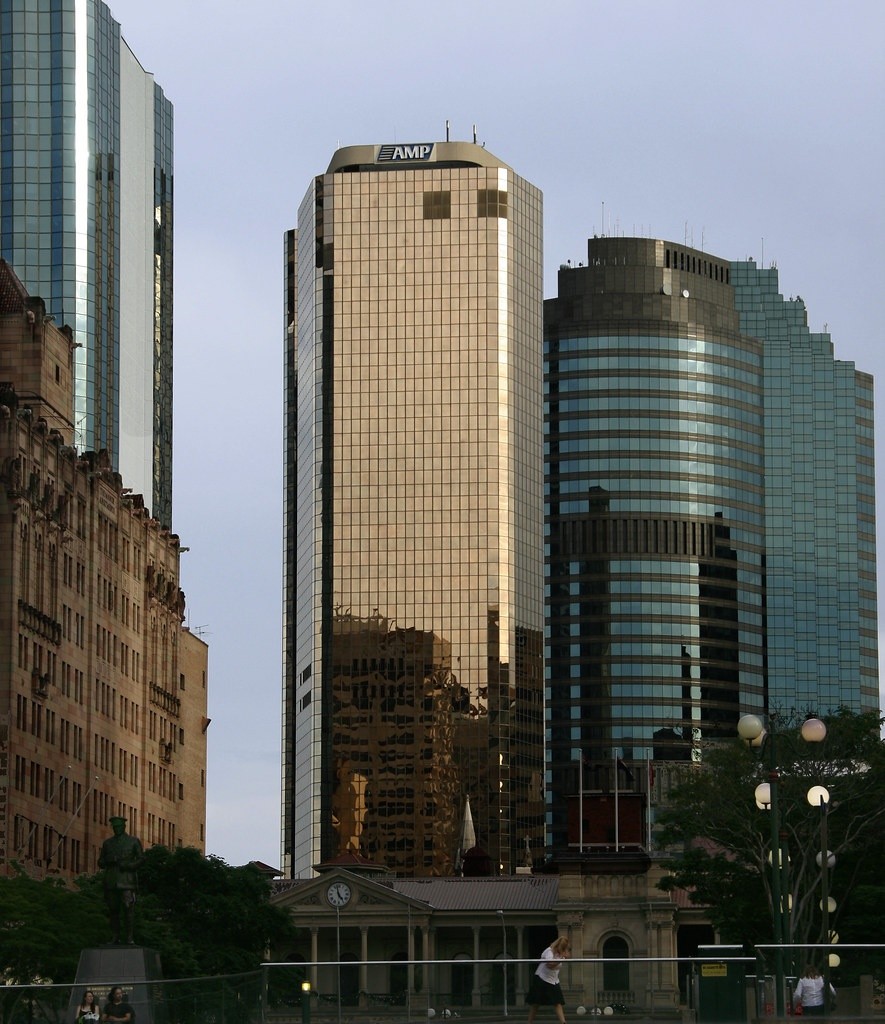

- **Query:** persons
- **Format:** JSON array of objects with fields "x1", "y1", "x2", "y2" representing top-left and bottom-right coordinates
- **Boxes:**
[
  {"x1": 793, "y1": 964, "x2": 837, "y2": 1024},
  {"x1": 74, "y1": 991, "x2": 100, "y2": 1024},
  {"x1": 526, "y1": 936, "x2": 572, "y2": 1024},
  {"x1": 96, "y1": 816, "x2": 147, "y2": 946},
  {"x1": 101, "y1": 985, "x2": 132, "y2": 1024},
  {"x1": 526, "y1": 850, "x2": 533, "y2": 867}
]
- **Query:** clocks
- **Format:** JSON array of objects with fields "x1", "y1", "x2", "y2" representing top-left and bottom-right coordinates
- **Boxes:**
[{"x1": 325, "y1": 880, "x2": 354, "y2": 910}]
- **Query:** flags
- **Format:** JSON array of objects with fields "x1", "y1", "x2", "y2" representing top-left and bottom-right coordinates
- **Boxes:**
[{"x1": 463, "y1": 798, "x2": 476, "y2": 854}]
